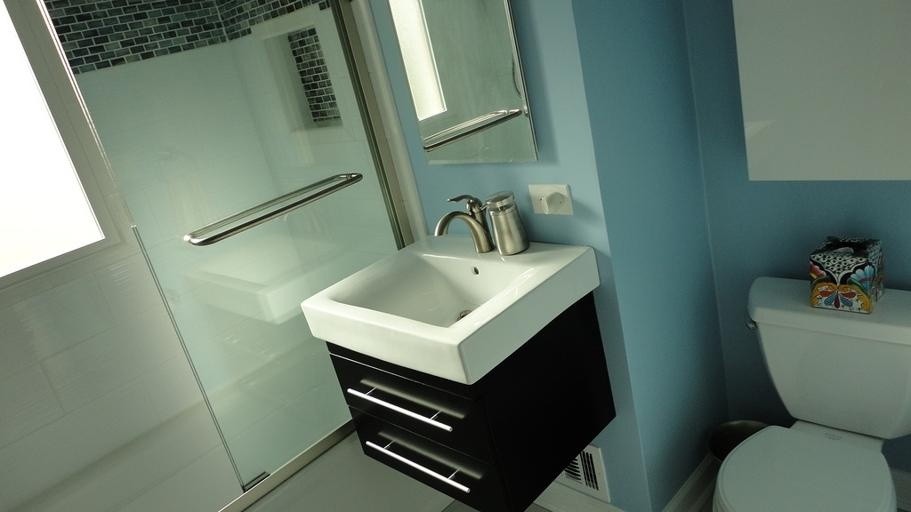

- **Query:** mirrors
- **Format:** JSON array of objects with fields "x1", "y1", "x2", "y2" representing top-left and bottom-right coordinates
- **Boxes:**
[{"x1": 389, "y1": 0, "x2": 541, "y2": 165}]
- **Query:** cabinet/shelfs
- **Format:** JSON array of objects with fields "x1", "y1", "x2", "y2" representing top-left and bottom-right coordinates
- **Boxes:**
[{"x1": 325, "y1": 290, "x2": 616, "y2": 511}]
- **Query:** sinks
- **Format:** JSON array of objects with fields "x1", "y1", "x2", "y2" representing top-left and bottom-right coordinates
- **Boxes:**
[
  {"x1": 299, "y1": 234, "x2": 603, "y2": 386},
  {"x1": 186, "y1": 235, "x2": 366, "y2": 324}
]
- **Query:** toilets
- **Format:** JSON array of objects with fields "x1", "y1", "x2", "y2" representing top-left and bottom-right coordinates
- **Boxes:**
[{"x1": 711, "y1": 277, "x2": 911, "y2": 512}]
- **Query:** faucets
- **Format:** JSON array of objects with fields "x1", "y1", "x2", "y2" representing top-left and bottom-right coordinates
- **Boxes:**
[
  {"x1": 278, "y1": 209, "x2": 325, "y2": 234},
  {"x1": 433, "y1": 193, "x2": 491, "y2": 254}
]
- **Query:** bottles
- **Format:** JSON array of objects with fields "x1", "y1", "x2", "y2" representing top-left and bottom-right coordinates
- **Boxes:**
[{"x1": 487, "y1": 190, "x2": 530, "y2": 257}]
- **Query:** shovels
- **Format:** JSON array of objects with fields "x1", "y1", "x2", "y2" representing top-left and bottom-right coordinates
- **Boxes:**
[{"x1": 706, "y1": 420, "x2": 769, "y2": 469}]
